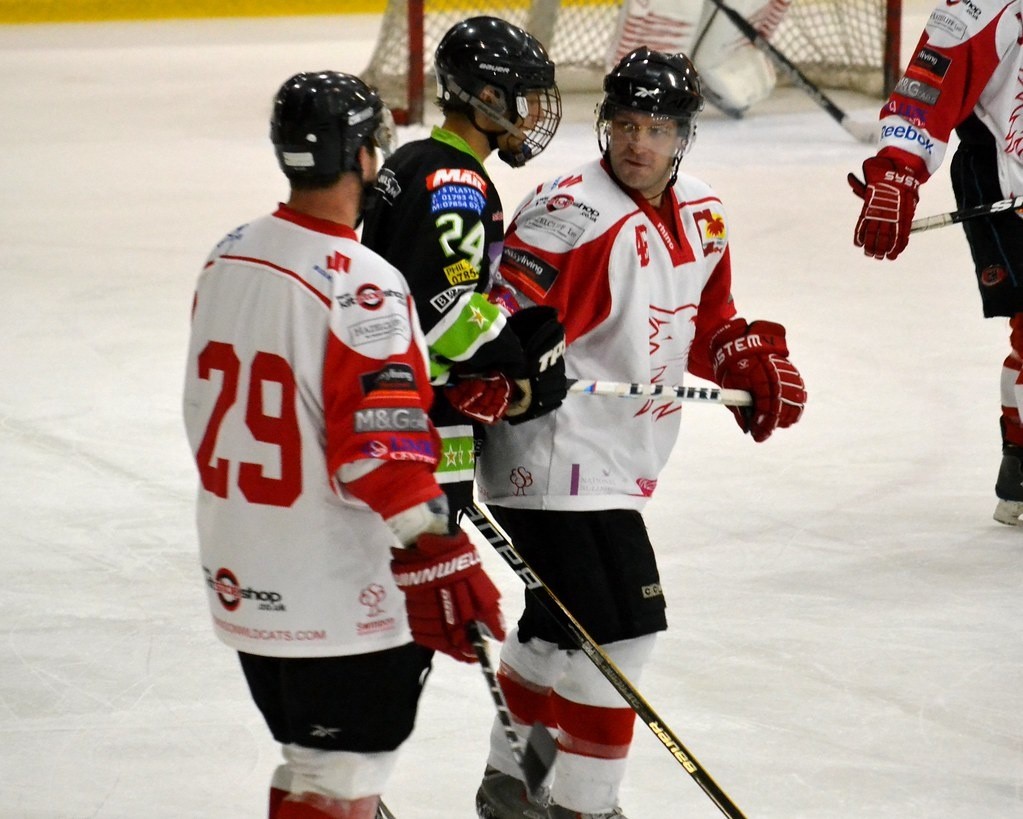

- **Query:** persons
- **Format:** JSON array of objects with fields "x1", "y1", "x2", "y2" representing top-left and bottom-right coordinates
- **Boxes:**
[
  {"x1": 847, "y1": 0, "x2": 1023, "y2": 529},
  {"x1": 445, "y1": 46, "x2": 809, "y2": 819},
  {"x1": 609, "y1": 0, "x2": 791, "y2": 119},
  {"x1": 181, "y1": 71, "x2": 509, "y2": 819},
  {"x1": 363, "y1": 14, "x2": 570, "y2": 527}
]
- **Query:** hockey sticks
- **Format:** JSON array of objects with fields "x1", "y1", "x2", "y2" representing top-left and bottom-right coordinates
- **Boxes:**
[
  {"x1": 454, "y1": 498, "x2": 746, "y2": 818},
  {"x1": 909, "y1": 195, "x2": 1023, "y2": 234},
  {"x1": 468, "y1": 623, "x2": 562, "y2": 798},
  {"x1": 713, "y1": 0, "x2": 880, "y2": 145},
  {"x1": 427, "y1": 368, "x2": 755, "y2": 412}
]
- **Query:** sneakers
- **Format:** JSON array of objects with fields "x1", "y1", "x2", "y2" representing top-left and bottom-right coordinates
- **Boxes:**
[
  {"x1": 992, "y1": 415, "x2": 1023, "y2": 526},
  {"x1": 477, "y1": 763, "x2": 628, "y2": 819}
]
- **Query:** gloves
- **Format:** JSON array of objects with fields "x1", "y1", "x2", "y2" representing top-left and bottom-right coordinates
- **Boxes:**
[
  {"x1": 390, "y1": 531, "x2": 506, "y2": 664},
  {"x1": 847, "y1": 156, "x2": 919, "y2": 261},
  {"x1": 708, "y1": 318, "x2": 807, "y2": 443},
  {"x1": 444, "y1": 372, "x2": 512, "y2": 425},
  {"x1": 478, "y1": 305, "x2": 567, "y2": 426}
]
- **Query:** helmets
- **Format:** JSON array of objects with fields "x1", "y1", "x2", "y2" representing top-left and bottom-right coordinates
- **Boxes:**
[
  {"x1": 436, "y1": 16, "x2": 562, "y2": 168},
  {"x1": 272, "y1": 70, "x2": 383, "y2": 182},
  {"x1": 598, "y1": 46, "x2": 703, "y2": 126}
]
